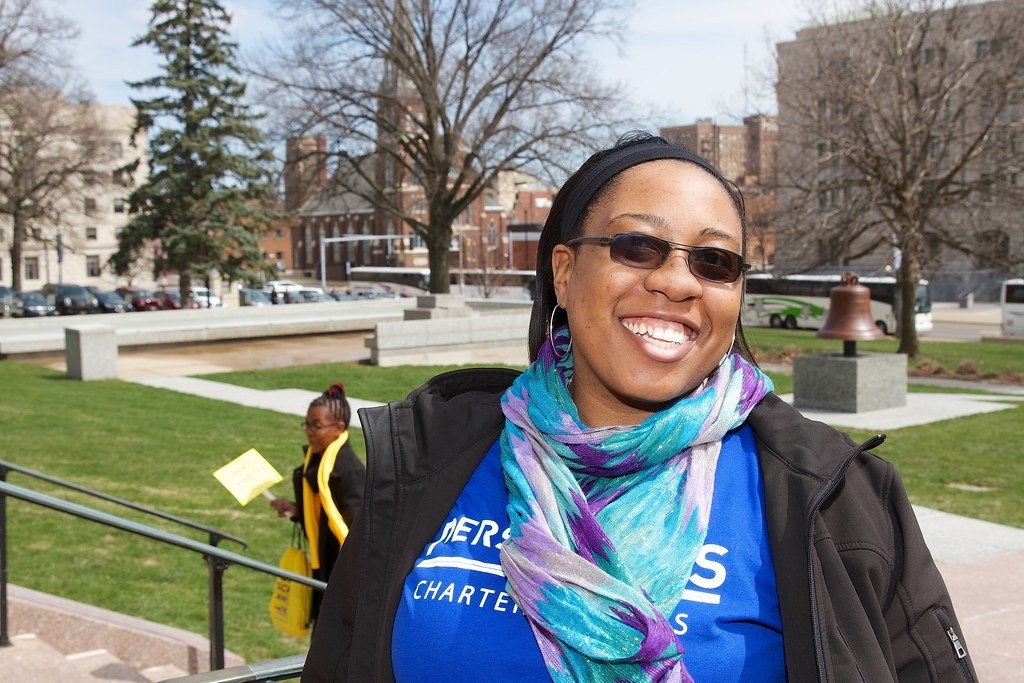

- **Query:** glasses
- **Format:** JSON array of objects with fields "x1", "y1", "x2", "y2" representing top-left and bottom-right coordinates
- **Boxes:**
[
  {"x1": 301, "y1": 421, "x2": 336, "y2": 433},
  {"x1": 566, "y1": 231, "x2": 752, "y2": 284}
]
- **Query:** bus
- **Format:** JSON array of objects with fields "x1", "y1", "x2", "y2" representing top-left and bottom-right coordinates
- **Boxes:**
[
  {"x1": 741, "y1": 273, "x2": 932, "y2": 335},
  {"x1": 1002, "y1": 278, "x2": 1024, "y2": 338}
]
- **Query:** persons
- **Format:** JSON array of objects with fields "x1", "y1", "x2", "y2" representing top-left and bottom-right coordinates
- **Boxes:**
[
  {"x1": 299, "y1": 128, "x2": 981, "y2": 682},
  {"x1": 268, "y1": 383, "x2": 365, "y2": 645}
]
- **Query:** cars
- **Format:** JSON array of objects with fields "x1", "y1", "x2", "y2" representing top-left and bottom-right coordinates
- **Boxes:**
[
  {"x1": 239, "y1": 281, "x2": 425, "y2": 306},
  {"x1": 0, "y1": 282, "x2": 224, "y2": 317}
]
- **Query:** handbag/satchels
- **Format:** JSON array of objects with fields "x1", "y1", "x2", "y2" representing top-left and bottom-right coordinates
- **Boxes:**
[{"x1": 269, "y1": 523, "x2": 313, "y2": 638}]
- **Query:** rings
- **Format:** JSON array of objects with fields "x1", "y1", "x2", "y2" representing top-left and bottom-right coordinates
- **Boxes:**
[{"x1": 278, "y1": 502, "x2": 282, "y2": 505}]
